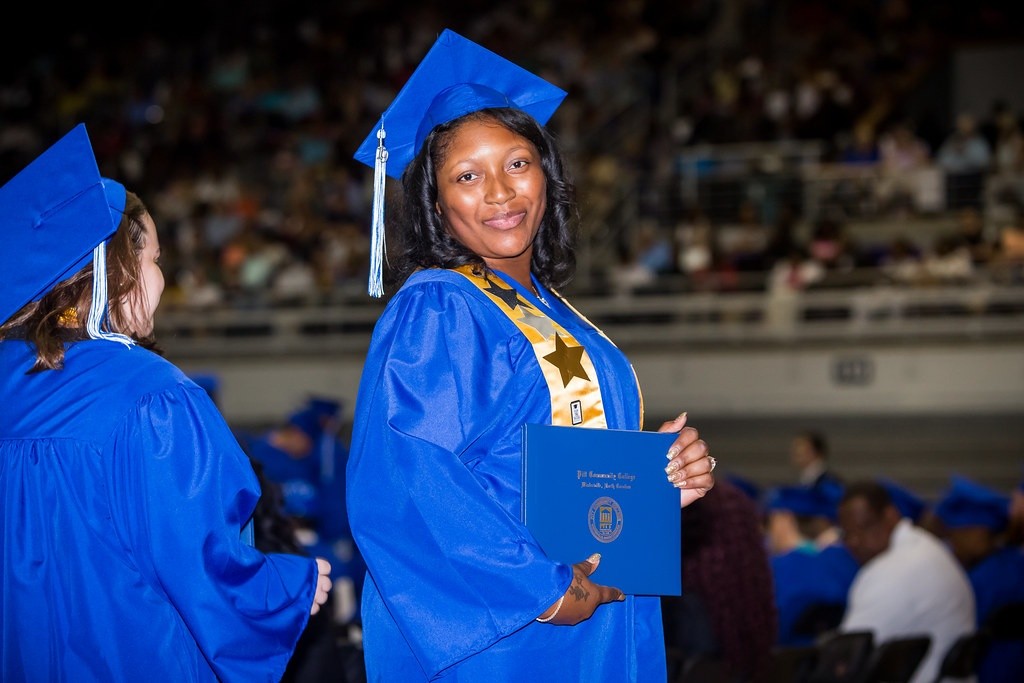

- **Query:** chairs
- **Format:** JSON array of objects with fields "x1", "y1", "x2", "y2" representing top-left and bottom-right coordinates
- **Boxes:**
[{"x1": 157, "y1": 142, "x2": 1022, "y2": 683}]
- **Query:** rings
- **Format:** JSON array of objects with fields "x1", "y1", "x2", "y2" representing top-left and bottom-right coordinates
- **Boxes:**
[{"x1": 707, "y1": 455, "x2": 717, "y2": 472}]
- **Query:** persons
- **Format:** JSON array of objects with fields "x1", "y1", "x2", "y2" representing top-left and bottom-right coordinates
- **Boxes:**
[
  {"x1": 332, "y1": 30, "x2": 716, "y2": 683},
  {"x1": 0, "y1": 122, "x2": 330, "y2": 683},
  {"x1": 29, "y1": 21, "x2": 1023, "y2": 321},
  {"x1": 256, "y1": 380, "x2": 1024, "y2": 565},
  {"x1": 829, "y1": 482, "x2": 979, "y2": 682}
]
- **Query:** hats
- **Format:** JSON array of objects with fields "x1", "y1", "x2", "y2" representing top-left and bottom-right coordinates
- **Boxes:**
[
  {"x1": 354, "y1": 33, "x2": 568, "y2": 298},
  {"x1": 1, "y1": 121, "x2": 138, "y2": 351},
  {"x1": 730, "y1": 472, "x2": 1012, "y2": 531},
  {"x1": 186, "y1": 373, "x2": 342, "y2": 442}
]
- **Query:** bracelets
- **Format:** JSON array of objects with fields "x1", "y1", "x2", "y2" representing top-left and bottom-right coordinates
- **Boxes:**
[{"x1": 533, "y1": 595, "x2": 565, "y2": 623}]
